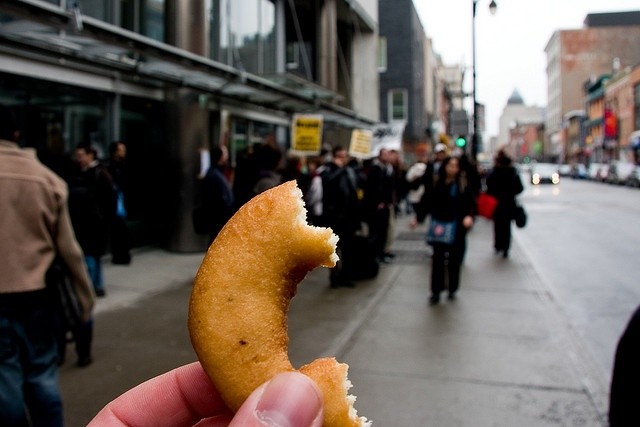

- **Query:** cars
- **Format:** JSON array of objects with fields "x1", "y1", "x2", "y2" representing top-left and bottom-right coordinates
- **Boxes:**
[
  {"x1": 632, "y1": 163, "x2": 640, "y2": 186},
  {"x1": 597, "y1": 167, "x2": 608, "y2": 181},
  {"x1": 531, "y1": 163, "x2": 559, "y2": 184},
  {"x1": 570, "y1": 163, "x2": 589, "y2": 178}
]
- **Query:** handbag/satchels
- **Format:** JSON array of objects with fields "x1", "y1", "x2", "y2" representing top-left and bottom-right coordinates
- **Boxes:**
[
  {"x1": 477, "y1": 190, "x2": 498, "y2": 218},
  {"x1": 46, "y1": 259, "x2": 95, "y2": 324},
  {"x1": 515, "y1": 201, "x2": 527, "y2": 228},
  {"x1": 425, "y1": 220, "x2": 455, "y2": 242}
]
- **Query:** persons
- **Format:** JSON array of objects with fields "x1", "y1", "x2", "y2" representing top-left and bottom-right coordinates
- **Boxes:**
[
  {"x1": 73, "y1": 144, "x2": 117, "y2": 297},
  {"x1": 306, "y1": 155, "x2": 322, "y2": 174},
  {"x1": 361, "y1": 148, "x2": 410, "y2": 265},
  {"x1": 50, "y1": 157, "x2": 95, "y2": 368},
  {"x1": 0, "y1": 95, "x2": 97, "y2": 426},
  {"x1": 86, "y1": 359, "x2": 326, "y2": 426},
  {"x1": 427, "y1": 141, "x2": 451, "y2": 184},
  {"x1": 281, "y1": 157, "x2": 306, "y2": 187},
  {"x1": 410, "y1": 156, "x2": 478, "y2": 305},
  {"x1": 609, "y1": 304, "x2": 640, "y2": 426},
  {"x1": 103, "y1": 142, "x2": 136, "y2": 264},
  {"x1": 486, "y1": 149, "x2": 523, "y2": 258},
  {"x1": 201, "y1": 144, "x2": 234, "y2": 250},
  {"x1": 251, "y1": 144, "x2": 286, "y2": 195},
  {"x1": 319, "y1": 145, "x2": 361, "y2": 288}
]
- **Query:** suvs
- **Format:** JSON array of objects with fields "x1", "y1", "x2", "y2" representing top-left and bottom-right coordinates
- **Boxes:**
[{"x1": 608, "y1": 160, "x2": 632, "y2": 186}]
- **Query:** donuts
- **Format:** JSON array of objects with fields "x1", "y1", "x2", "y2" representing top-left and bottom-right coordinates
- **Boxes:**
[{"x1": 188, "y1": 177, "x2": 373, "y2": 426}]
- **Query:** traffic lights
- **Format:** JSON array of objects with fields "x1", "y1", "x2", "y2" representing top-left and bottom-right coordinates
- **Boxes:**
[{"x1": 456, "y1": 136, "x2": 466, "y2": 147}]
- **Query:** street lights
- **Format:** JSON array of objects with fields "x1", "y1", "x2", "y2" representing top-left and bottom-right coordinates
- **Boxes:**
[{"x1": 472, "y1": 0, "x2": 497, "y2": 169}]
